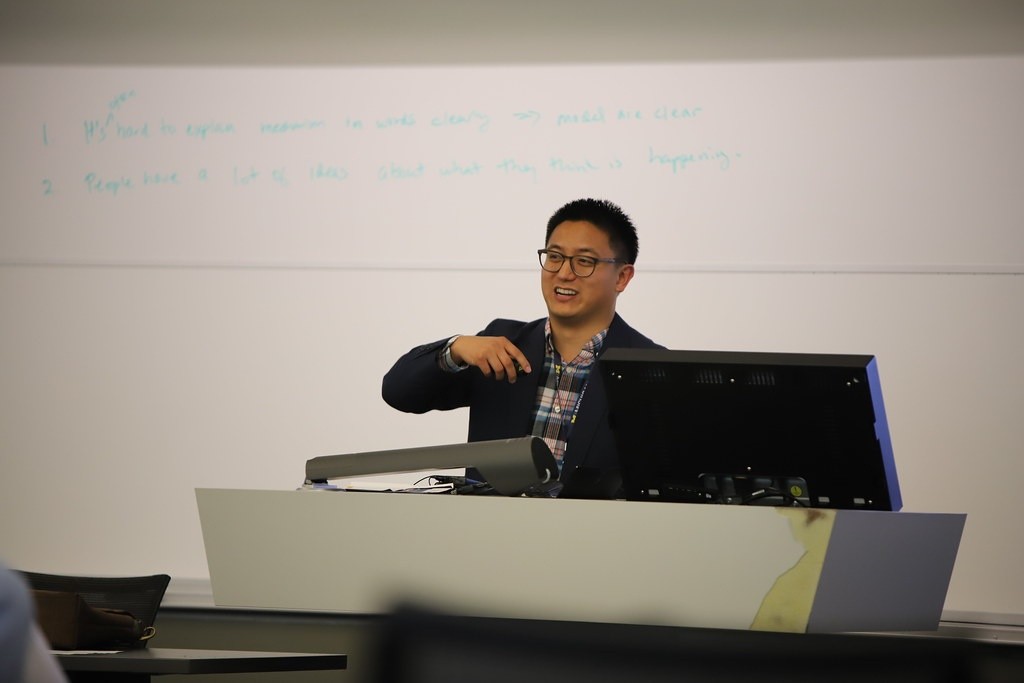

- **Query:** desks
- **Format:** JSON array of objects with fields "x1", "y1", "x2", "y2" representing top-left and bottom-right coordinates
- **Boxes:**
[{"x1": 52, "y1": 647, "x2": 347, "y2": 683}]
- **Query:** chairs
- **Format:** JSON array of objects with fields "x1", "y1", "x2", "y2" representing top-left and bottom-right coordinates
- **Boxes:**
[{"x1": 5, "y1": 568, "x2": 170, "y2": 651}]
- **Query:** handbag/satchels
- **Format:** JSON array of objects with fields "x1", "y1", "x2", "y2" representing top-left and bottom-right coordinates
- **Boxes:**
[{"x1": 36, "y1": 594, "x2": 156, "y2": 650}]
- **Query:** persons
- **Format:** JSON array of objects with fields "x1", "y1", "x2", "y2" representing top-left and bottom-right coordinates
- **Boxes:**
[{"x1": 383, "y1": 199, "x2": 671, "y2": 495}]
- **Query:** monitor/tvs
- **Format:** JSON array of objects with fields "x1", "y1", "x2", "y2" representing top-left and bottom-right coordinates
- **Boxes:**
[{"x1": 600, "y1": 347, "x2": 904, "y2": 511}]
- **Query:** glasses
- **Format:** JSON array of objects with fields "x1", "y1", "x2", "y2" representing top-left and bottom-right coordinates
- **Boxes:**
[{"x1": 537, "y1": 249, "x2": 625, "y2": 278}]
